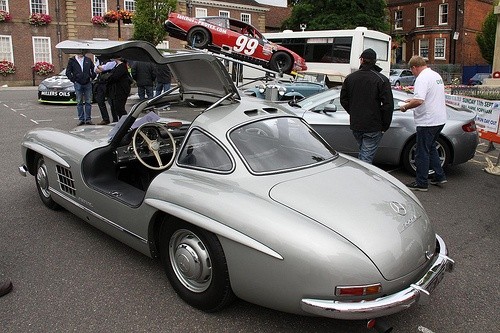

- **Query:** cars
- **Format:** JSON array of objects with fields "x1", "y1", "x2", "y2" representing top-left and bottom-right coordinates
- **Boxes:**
[
  {"x1": 243, "y1": 84, "x2": 479, "y2": 177},
  {"x1": 390, "y1": 68, "x2": 416, "y2": 87},
  {"x1": 36, "y1": 68, "x2": 108, "y2": 106},
  {"x1": 164, "y1": 11, "x2": 308, "y2": 75},
  {"x1": 18, "y1": 38, "x2": 456, "y2": 333}
]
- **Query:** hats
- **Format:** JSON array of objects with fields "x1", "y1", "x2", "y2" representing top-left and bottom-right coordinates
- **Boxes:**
[{"x1": 359, "y1": 48, "x2": 377, "y2": 60}]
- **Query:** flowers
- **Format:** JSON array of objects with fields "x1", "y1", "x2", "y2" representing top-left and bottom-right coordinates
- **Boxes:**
[
  {"x1": 31, "y1": 61, "x2": 55, "y2": 76},
  {"x1": 0, "y1": 9, "x2": 12, "y2": 23},
  {"x1": 118, "y1": 9, "x2": 133, "y2": 19},
  {"x1": 27, "y1": 12, "x2": 52, "y2": 27},
  {"x1": 0, "y1": 60, "x2": 17, "y2": 77},
  {"x1": 102, "y1": 9, "x2": 119, "y2": 20},
  {"x1": 91, "y1": 16, "x2": 109, "y2": 27}
]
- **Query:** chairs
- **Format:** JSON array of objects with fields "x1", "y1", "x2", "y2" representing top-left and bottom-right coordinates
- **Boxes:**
[
  {"x1": 213, "y1": 162, "x2": 232, "y2": 171},
  {"x1": 244, "y1": 148, "x2": 279, "y2": 171}
]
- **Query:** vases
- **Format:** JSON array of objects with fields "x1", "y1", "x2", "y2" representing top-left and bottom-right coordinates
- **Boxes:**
[
  {"x1": 104, "y1": 15, "x2": 117, "y2": 23},
  {"x1": 122, "y1": 16, "x2": 133, "y2": 24}
]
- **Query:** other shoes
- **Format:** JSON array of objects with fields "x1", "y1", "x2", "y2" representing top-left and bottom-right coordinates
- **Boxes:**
[
  {"x1": 85, "y1": 121, "x2": 95, "y2": 125},
  {"x1": 77, "y1": 120, "x2": 85, "y2": 126},
  {"x1": 0, "y1": 280, "x2": 13, "y2": 296},
  {"x1": 406, "y1": 181, "x2": 429, "y2": 192},
  {"x1": 113, "y1": 119, "x2": 118, "y2": 122},
  {"x1": 429, "y1": 176, "x2": 448, "y2": 185},
  {"x1": 97, "y1": 120, "x2": 110, "y2": 125}
]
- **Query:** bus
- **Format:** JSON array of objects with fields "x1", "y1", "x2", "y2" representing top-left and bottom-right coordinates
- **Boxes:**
[{"x1": 223, "y1": 23, "x2": 392, "y2": 85}]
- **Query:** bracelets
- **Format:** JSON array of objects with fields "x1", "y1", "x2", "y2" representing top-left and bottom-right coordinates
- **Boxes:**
[{"x1": 403, "y1": 106, "x2": 407, "y2": 111}]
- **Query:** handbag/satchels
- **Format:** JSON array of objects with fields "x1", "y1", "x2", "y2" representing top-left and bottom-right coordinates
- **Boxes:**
[{"x1": 484, "y1": 157, "x2": 500, "y2": 176}]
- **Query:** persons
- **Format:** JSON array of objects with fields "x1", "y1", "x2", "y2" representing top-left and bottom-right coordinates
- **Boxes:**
[
  {"x1": 94, "y1": 54, "x2": 133, "y2": 126},
  {"x1": 0, "y1": 279, "x2": 14, "y2": 297},
  {"x1": 400, "y1": 56, "x2": 448, "y2": 192},
  {"x1": 131, "y1": 59, "x2": 155, "y2": 100},
  {"x1": 154, "y1": 63, "x2": 172, "y2": 98},
  {"x1": 66, "y1": 52, "x2": 96, "y2": 126},
  {"x1": 180, "y1": 146, "x2": 197, "y2": 166},
  {"x1": 340, "y1": 48, "x2": 394, "y2": 165}
]
